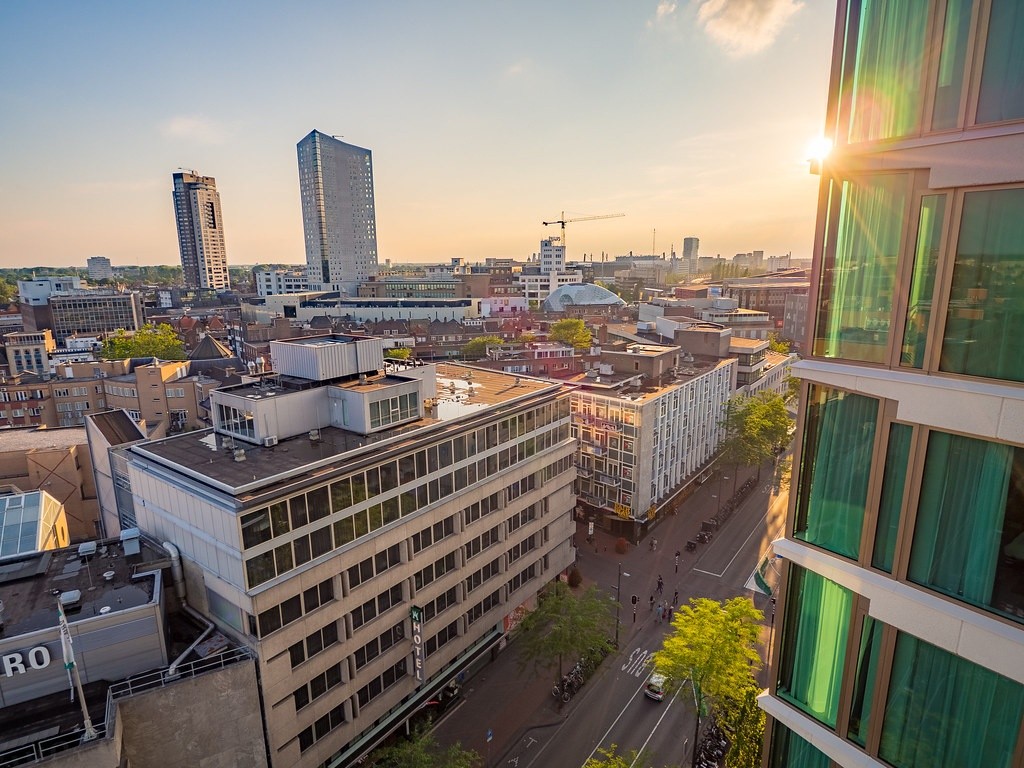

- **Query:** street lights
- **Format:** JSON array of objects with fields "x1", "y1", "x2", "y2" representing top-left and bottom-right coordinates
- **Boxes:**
[
  {"x1": 715, "y1": 476, "x2": 730, "y2": 532},
  {"x1": 608, "y1": 562, "x2": 631, "y2": 645}
]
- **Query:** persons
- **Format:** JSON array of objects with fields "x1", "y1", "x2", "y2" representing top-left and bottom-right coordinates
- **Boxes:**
[
  {"x1": 651, "y1": 538, "x2": 657, "y2": 551},
  {"x1": 646, "y1": 574, "x2": 679, "y2": 623},
  {"x1": 573, "y1": 542, "x2": 577, "y2": 548}
]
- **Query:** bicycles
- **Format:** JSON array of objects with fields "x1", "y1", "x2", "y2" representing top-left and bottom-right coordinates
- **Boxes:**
[
  {"x1": 694, "y1": 715, "x2": 728, "y2": 768},
  {"x1": 551, "y1": 636, "x2": 620, "y2": 703},
  {"x1": 684, "y1": 475, "x2": 758, "y2": 554}
]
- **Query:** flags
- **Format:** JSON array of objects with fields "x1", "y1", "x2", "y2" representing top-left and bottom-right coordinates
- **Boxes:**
[{"x1": 58, "y1": 599, "x2": 77, "y2": 704}]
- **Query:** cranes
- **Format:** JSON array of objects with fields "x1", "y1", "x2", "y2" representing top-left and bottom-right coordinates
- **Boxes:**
[{"x1": 542, "y1": 211, "x2": 627, "y2": 246}]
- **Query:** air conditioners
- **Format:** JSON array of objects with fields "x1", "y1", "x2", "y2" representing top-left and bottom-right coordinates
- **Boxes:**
[{"x1": 264, "y1": 436, "x2": 278, "y2": 446}]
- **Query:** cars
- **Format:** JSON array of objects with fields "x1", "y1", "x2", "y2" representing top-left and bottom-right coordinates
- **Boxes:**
[{"x1": 644, "y1": 669, "x2": 674, "y2": 702}]
